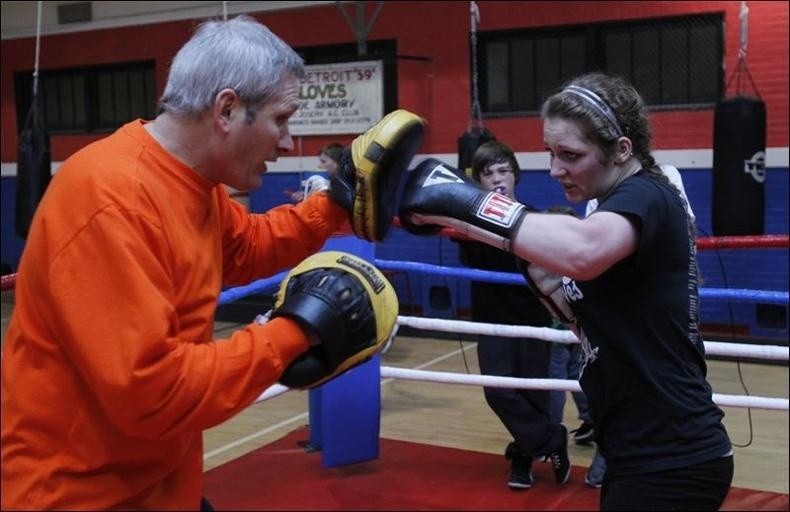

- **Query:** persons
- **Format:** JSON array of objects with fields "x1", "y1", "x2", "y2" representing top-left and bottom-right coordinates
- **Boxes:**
[
  {"x1": 452, "y1": 138, "x2": 573, "y2": 492},
  {"x1": 399, "y1": 70, "x2": 736, "y2": 510},
  {"x1": 2, "y1": 11, "x2": 429, "y2": 511},
  {"x1": 536, "y1": 204, "x2": 599, "y2": 443},
  {"x1": 584, "y1": 163, "x2": 698, "y2": 488}
]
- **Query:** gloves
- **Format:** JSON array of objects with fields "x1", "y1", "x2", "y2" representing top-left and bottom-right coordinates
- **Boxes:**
[
  {"x1": 511, "y1": 205, "x2": 597, "y2": 327},
  {"x1": 255, "y1": 250, "x2": 402, "y2": 391},
  {"x1": 397, "y1": 154, "x2": 529, "y2": 255},
  {"x1": 326, "y1": 106, "x2": 428, "y2": 243}
]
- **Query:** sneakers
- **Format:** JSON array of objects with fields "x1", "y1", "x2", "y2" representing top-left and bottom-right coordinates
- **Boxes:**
[
  {"x1": 506, "y1": 441, "x2": 534, "y2": 489},
  {"x1": 575, "y1": 419, "x2": 594, "y2": 444},
  {"x1": 550, "y1": 423, "x2": 572, "y2": 485},
  {"x1": 585, "y1": 449, "x2": 606, "y2": 487}
]
